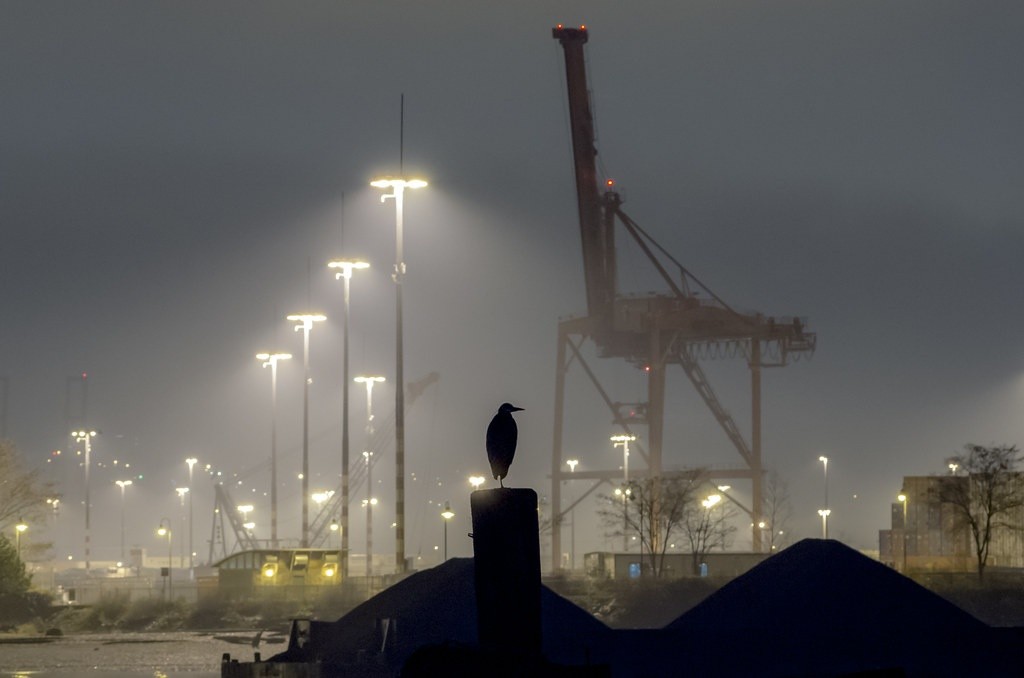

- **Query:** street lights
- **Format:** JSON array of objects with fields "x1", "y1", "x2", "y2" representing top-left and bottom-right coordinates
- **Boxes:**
[
  {"x1": 175, "y1": 487, "x2": 189, "y2": 567},
  {"x1": 16, "y1": 524, "x2": 27, "y2": 562},
  {"x1": 610, "y1": 435, "x2": 636, "y2": 551},
  {"x1": 567, "y1": 459, "x2": 580, "y2": 570},
  {"x1": 157, "y1": 518, "x2": 172, "y2": 601},
  {"x1": 899, "y1": 496, "x2": 907, "y2": 574},
  {"x1": 820, "y1": 455, "x2": 828, "y2": 510},
  {"x1": 353, "y1": 376, "x2": 385, "y2": 576},
  {"x1": 328, "y1": 260, "x2": 370, "y2": 583},
  {"x1": 818, "y1": 509, "x2": 832, "y2": 537},
  {"x1": 46, "y1": 498, "x2": 59, "y2": 572},
  {"x1": 115, "y1": 480, "x2": 132, "y2": 578},
  {"x1": 256, "y1": 352, "x2": 292, "y2": 549},
  {"x1": 70, "y1": 427, "x2": 96, "y2": 581},
  {"x1": 370, "y1": 179, "x2": 427, "y2": 572},
  {"x1": 440, "y1": 500, "x2": 454, "y2": 563},
  {"x1": 718, "y1": 486, "x2": 730, "y2": 551},
  {"x1": 286, "y1": 314, "x2": 327, "y2": 548},
  {"x1": 186, "y1": 458, "x2": 198, "y2": 577}
]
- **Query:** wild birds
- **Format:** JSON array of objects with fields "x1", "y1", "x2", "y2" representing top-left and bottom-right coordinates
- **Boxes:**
[{"x1": 486, "y1": 402, "x2": 526, "y2": 488}]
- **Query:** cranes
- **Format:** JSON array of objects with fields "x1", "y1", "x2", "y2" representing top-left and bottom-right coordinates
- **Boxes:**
[
  {"x1": 208, "y1": 371, "x2": 439, "y2": 567},
  {"x1": 544, "y1": 25, "x2": 819, "y2": 575}
]
